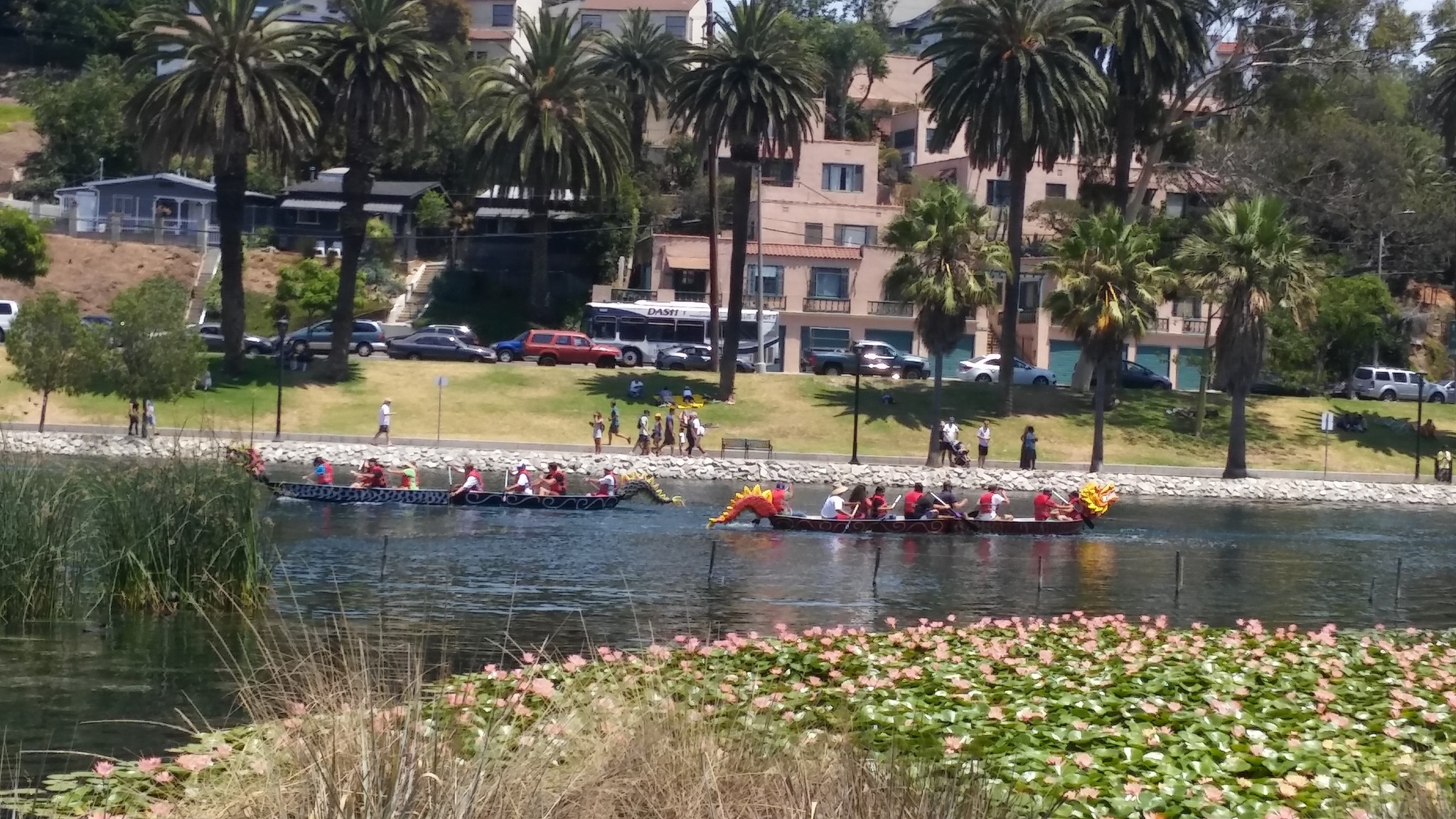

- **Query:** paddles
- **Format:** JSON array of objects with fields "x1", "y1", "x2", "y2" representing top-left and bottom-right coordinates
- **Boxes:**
[
  {"x1": 1051, "y1": 490, "x2": 1095, "y2": 530},
  {"x1": 585, "y1": 478, "x2": 600, "y2": 491},
  {"x1": 502, "y1": 467, "x2": 509, "y2": 507},
  {"x1": 842, "y1": 504, "x2": 860, "y2": 533},
  {"x1": 886, "y1": 493, "x2": 902, "y2": 514},
  {"x1": 929, "y1": 491, "x2": 980, "y2": 533},
  {"x1": 447, "y1": 465, "x2": 456, "y2": 513}
]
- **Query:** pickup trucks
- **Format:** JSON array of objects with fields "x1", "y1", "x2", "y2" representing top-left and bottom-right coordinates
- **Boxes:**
[{"x1": 811, "y1": 340, "x2": 931, "y2": 380}]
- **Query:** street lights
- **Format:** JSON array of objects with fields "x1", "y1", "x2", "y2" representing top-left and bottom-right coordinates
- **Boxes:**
[
  {"x1": 849, "y1": 343, "x2": 867, "y2": 464},
  {"x1": 272, "y1": 319, "x2": 289, "y2": 443},
  {"x1": 1415, "y1": 370, "x2": 1428, "y2": 481}
]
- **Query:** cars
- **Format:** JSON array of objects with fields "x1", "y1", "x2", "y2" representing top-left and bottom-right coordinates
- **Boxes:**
[
  {"x1": 1071, "y1": 360, "x2": 1172, "y2": 389},
  {"x1": 1250, "y1": 370, "x2": 1310, "y2": 397},
  {"x1": 387, "y1": 333, "x2": 497, "y2": 363},
  {"x1": 1433, "y1": 380, "x2": 1456, "y2": 404},
  {"x1": 187, "y1": 322, "x2": 275, "y2": 355},
  {"x1": 655, "y1": 345, "x2": 755, "y2": 373},
  {"x1": 957, "y1": 353, "x2": 1057, "y2": 386}
]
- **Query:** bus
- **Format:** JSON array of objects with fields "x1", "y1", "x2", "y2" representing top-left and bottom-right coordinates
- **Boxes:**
[{"x1": 581, "y1": 300, "x2": 780, "y2": 368}]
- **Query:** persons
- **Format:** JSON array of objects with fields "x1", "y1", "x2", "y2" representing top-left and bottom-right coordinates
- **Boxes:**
[
  {"x1": 1057, "y1": 490, "x2": 1085, "y2": 520},
  {"x1": 1019, "y1": 425, "x2": 1038, "y2": 470},
  {"x1": 934, "y1": 480, "x2": 968, "y2": 517},
  {"x1": 1337, "y1": 412, "x2": 1368, "y2": 433},
  {"x1": 769, "y1": 482, "x2": 806, "y2": 517},
  {"x1": 977, "y1": 420, "x2": 991, "y2": 470},
  {"x1": 192, "y1": 366, "x2": 213, "y2": 390},
  {"x1": 848, "y1": 483, "x2": 873, "y2": 519},
  {"x1": 903, "y1": 482, "x2": 950, "y2": 519},
  {"x1": 882, "y1": 392, "x2": 894, "y2": 406},
  {"x1": 1033, "y1": 487, "x2": 1074, "y2": 520},
  {"x1": 937, "y1": 416, "x2": 971, "y2": 468},
  {"x1": 819, "y1": 481, "x2": 861, "y2": 519},
  {"x1": 301, "y1": 455, "x2": 618, "y2": 496},
  {"x1": 371, "y1": 399, "x2": 397, "y2": 445},
  {"x1": 975, "y1": 483, "x2": 1013, "y2": 520},
  {"x1": 870, "y1": 485, "x2": 897, "y2": 519},
  {"x1": 586, "y1": 376, "x2": 708, "y2": 458},
  {"x1": 128, "y1": 392, "x2": 159, "y2": 436},
  {"x1": 1421, "y1": 419, "x2": 1453, "y2": 482}
]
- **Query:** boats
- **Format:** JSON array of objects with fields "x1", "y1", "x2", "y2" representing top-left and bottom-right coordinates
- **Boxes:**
[
  {"x1": 225, "y1": 446, "x2": 686, "y2": 510},
  {"x1": 709, "y1": 481, "x2": 1119, "y2": 535}
]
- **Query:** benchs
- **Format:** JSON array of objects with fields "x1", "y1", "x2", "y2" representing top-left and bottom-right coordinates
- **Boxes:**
[{"x1": 720, "y1": 437, "x2": 772, "y2": 460}]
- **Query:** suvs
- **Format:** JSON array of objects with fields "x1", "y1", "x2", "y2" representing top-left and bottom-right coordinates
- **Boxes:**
[
  {"x1": 490, "y1": 332, "x2": 526, "y2": 363},
  {"x1": 280, "y1": 319, "x2": 388, "y2": 357},
  {"x1": 1353, "y1": 366, "x2": 1448, "y2": 405},
  {"x1": 0, "y1": 299, "x2": 20, "y2": 342},
  {"x1": 384, "y1": 324, "x2": 481, "y2": 352},
  {"x1": 523, "y1": 329, "x2": 620, "y2": 369}
]
